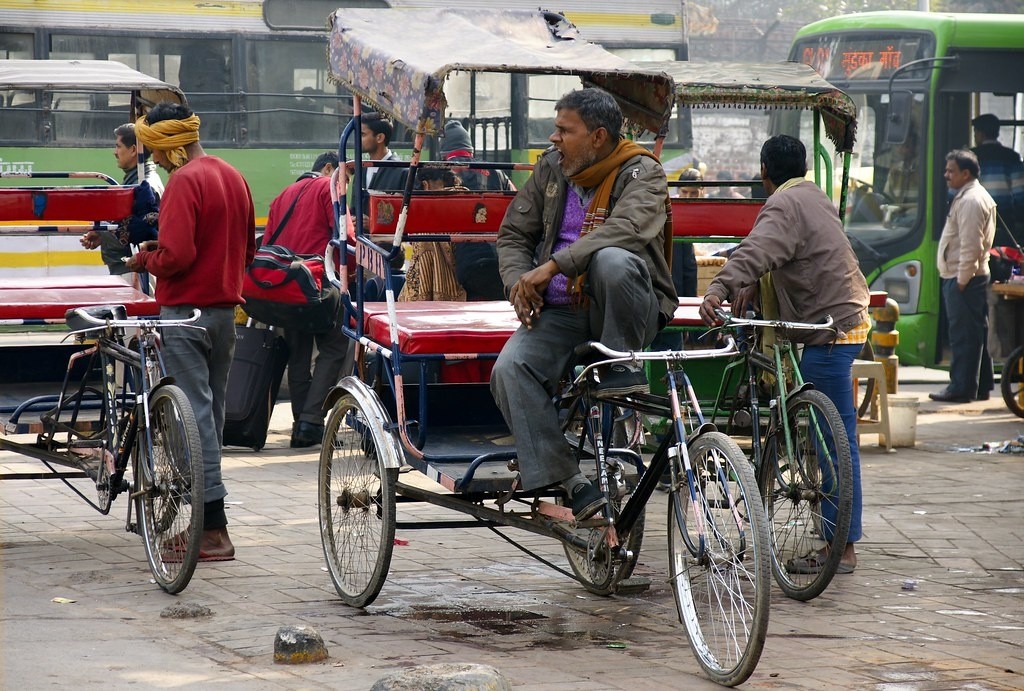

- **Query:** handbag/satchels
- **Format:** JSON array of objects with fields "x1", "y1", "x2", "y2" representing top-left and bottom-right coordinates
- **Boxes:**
[{"x1": 242, "y1": 243, "x2": 326, "y2": 305}]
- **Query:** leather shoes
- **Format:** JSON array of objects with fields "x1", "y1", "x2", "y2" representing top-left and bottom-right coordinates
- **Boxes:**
[{"x1": 289, "y1": 420, "x2": 345, "y2": 448}]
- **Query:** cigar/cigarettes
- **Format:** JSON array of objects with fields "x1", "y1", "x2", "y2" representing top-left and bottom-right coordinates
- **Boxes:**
[{"x1": 530, "y1": 310, "x2": 534, "y2": 316}]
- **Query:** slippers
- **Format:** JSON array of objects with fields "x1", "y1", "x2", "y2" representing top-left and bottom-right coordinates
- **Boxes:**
[
  {"x1": 161, "y1": 547, "x2": 235, "y2": 563},
  {"x1": 164, "y1": 531, "x2": 189, "y2": 551},
  {"x1": 783, "y1": 547, "x2": 855, "y2": 575}
]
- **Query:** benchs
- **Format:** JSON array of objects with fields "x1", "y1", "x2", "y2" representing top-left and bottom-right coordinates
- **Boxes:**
[
  {"x1": 329, "y1": 161, "x2": 776, "y2": 443},
  {"x1": 0, "y1": 171, "x2": 158, "y2": 318}
]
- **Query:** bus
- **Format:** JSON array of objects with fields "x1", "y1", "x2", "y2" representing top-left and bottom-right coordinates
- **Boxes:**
[
  {"x1": 768, "y1": 11, "x2": 1024, "y2": 374},
  {"x1": 0, "y1": 0, "x2": 694, "y2": 394}
]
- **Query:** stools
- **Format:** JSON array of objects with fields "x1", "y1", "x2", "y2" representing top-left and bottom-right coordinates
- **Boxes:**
[{"x1": 854, "y1": 358, "x2": 893, "y2": 452}]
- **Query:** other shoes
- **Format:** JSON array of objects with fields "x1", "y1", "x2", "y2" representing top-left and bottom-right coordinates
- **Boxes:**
[
  {"x1": 977, "y1": 388, "x2": 990, "y2": 401},
  {"x1": 929, "y1": 386, "x2": 970, "y2": 403},
  {"x1": 569, "y1": 480, "x2": 609, "y2": 522},
  {"x1": 593, "y1": 365, "x2": 651, "y2": 398}
]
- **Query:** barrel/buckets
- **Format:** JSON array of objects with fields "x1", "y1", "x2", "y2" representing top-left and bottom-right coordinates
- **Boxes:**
[{"x1": 877, "y1": 393, "x2": 920, "y2": 448}]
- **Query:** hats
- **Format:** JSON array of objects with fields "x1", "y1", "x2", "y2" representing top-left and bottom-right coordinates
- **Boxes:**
[
  {"x1": 971, "y1": 114, "x2": 1000, "y2": 130},
  {"x1": 437, "y1": 120, "x2": 474, "y2": 157}
]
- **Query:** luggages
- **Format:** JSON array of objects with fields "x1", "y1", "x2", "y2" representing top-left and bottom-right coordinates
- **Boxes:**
[{"x1": 220, "y1": 315, "x2": 289, "y2": 452}]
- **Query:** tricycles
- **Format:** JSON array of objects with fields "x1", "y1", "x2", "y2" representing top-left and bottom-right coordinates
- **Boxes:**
[
  {"x1": 620, "y1": 57, "x2": 858, "y2": 597},
  {"x1": 311, "y1": 9, "x2": 772, "y2": 687},
  {"x1": 0, "y1": 56, "x2": 211, "y2": 595},
  {"x1": 986, "y1": 243, "x2": 1024, "y2": 416}
]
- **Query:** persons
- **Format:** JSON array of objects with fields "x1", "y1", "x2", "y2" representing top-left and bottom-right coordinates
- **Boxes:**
[
  {"x1": 733, "y1": 171, "x2": 751, "y2": 192},
  {"x1": 884, "y1": 132, "x2": 918, "y2": 226},
  {"x1": 489, "y1": 88, "x2": 679, "y2": 520},
  {"x1": 261, "y1": 148, "x2": 351, "y2": 449},
  {"x1": 80, "y1": 121, "x2": 165, "y2": 445},
  {"x1": 698, "y1": 133, "x2": 872, "y2": 574},
  {"x1": 709, "y1": 170, "x2": 747, "y2": 199},
  {"x1": 928, "y1": 149, "x2": 996, "y2": 403},
  {"x1": 946, "y1": 113, "x2": 1024, "y2": 362},
  {"x1": 352, "y1": 113, "x2": 409, "y2": 232},
  {"x1": 179, "y1": 40, "x2": 226, "y2": 141},
  {"x1": 365, "y1": 242, "x2": 408, "y2": 301},
  {"x1": 395, "y1": 167, "x2": 485, "y2": 302},
  {"x1": 440, "y1": 122, "x2": 518, "y2": 191},
  {"x1": 670, "y1": 167, "x2": 708, "y2": 198},
  {"x1": 125, "y1": 100, "x2": 255, "y2": 562}
]
